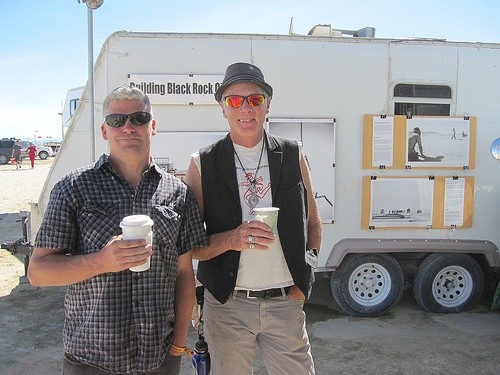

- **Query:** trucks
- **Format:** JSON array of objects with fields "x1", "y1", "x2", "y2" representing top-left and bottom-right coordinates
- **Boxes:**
[{"x1": 0, "y1": 28, "x2": 500, "y2": 318}]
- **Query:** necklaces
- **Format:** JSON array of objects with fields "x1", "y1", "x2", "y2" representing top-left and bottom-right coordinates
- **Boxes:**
[{"x1": 233, "y1": 136, "x2": 267, "y2": 207}]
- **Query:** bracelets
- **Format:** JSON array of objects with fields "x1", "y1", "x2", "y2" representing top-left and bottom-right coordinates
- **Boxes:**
[{"x1": 170, "y1": 345, "x2": 193, "y2": 359}]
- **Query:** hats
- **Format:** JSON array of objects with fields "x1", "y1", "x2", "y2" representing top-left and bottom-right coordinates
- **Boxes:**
[{"x1": 215, "y1": 63, "x2": 272, "y2": 103}]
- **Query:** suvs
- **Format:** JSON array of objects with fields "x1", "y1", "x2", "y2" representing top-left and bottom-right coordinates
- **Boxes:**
[{"x1": 0, "y1": 138, "x2": 54, "y2": 165}]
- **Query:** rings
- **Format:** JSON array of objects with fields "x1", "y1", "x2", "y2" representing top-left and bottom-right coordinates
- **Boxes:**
[
  {"x1": 247, "y1": 235, "x2": 254, "y2": 243},
  {"x1": 249, "y1": 243, "x2": 254, "y2": 249}
]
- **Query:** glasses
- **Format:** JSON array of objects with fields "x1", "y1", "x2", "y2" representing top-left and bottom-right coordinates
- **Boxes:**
[
  {"x1": 105, "y1": 111, "x2": 151, "y2": 127},
  {"x1": 223, "y1": 94, "x2": 268, "y2": 108}
]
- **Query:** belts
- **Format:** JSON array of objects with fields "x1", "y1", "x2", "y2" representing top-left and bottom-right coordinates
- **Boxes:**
[{"x1": 234, "y1": 284, "x2": 294, "y2": 299}]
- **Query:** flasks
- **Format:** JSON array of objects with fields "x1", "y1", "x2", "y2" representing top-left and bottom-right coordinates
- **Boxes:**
[{"x1": 192, "y1": 335, "x2": 210, "y2": 375}]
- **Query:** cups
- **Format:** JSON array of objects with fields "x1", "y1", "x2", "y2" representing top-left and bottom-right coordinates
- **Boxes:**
[
  {"x1": 253, "y1": 207, "x2": 280, "y2": 234},
  {"x1": 120, "y1": 214, "x2": 154, "y2": 272}
]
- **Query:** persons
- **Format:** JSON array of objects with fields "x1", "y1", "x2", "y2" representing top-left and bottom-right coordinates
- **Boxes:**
[
  {"x1": 28, "y1": 86, "x2": 197, "y2": 375},
  {"x1": 25, "y1": 141, "x2": 37, "y2": 169},
  {"x1": 182, "y1": 63, "x2": 323, "y2": 375},
  {"x1": 12, "y1": 141, "x2": 22, "y2": 170},
  {"x1": 450, "y1": 127, "x2": 457, "y2": 140},
  {"x1": 408, "y1": 127, "x2": 425, "y2": 162}
]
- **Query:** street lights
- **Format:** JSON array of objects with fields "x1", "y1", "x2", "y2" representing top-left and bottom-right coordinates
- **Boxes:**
[{"x1": 76, "y1": 0, "x2": 106, "y2": 163}]
